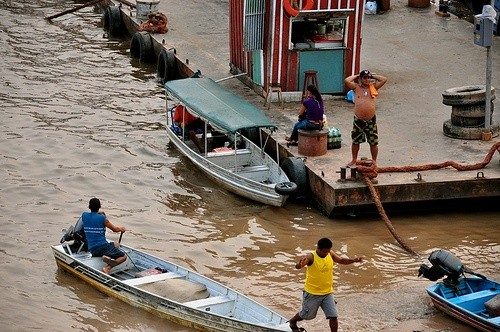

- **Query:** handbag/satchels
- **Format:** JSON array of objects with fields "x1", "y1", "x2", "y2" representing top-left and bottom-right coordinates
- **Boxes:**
[{"x1": 299, "y1": 112, "x2": 306, "y2": 121}]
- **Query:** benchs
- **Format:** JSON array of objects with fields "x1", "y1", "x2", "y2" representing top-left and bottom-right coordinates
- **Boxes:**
[
  {"x1": 72, "y1": 247, "x2": 133, "y2": 275},
  {"x1": 170, "y1": 106, "x2": 204, "y2": 135}
]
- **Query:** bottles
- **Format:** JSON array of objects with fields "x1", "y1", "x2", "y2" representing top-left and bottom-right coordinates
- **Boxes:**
[{"x1": 326, "y1": 129, "x2": 341, "y2": 150}]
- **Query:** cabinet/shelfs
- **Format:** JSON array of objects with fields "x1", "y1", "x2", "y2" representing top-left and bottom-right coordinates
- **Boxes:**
[{"x1": 295, "y1": 46, "x2": 348, "y2": 93}]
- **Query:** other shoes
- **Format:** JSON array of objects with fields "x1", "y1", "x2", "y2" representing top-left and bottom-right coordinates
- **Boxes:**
[
  {"x1": 285, "y1": 136, "x2": 298, "y2": 143},
  {"x1": 102, "y1": 267, "x2": 109, "y2": 275},
  {"x1": 102, "y1": 255, "x2": 116, "y2": 267},
  {"x1": 289, "y1": 325, "x2": 299, "y2": 332},
  {"x1": 347, "y1": 161, "x2": 356, "y2": 167}
]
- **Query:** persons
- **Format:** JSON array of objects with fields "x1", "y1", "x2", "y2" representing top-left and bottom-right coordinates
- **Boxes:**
[
  {"x1": 285, "y1": 85, "x2": 324, "y2": 143},
  {"x1": 82, "y1": 198, "x2": 127, "y2": 275},
  {"x1": 344, "y1": 70, "x2": 387, "y2": 165},
  {"x1": 289, "y1": 237, "x2": 366, "y2": 332},
  {"x1": 174, "y1": 102, "x2": 197, "y2": 140}
]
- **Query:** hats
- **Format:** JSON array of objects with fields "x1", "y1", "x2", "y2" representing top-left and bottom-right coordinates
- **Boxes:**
[{"x1": 360, "y1": 70, "x2": 372, "y2": 78}]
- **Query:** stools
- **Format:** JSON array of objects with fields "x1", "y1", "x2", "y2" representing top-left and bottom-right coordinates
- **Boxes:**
[
  {"x1": 264, "y1": 86, "x2": 285, "y2": 110},
  {"x1": 301, "y1": 70, "x2": 319, "y2": 104}
]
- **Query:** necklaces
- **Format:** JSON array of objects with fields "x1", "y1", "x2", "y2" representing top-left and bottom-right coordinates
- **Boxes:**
[{"x1": 361, "y1": 85, "x2": 370, "y2": 96}]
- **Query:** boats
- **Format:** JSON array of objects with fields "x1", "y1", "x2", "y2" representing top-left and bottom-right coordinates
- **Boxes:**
[
  {"x1": 50, "y1": 235, "x2": 299, "y2": 332},
  {"x1": 419, "y1": 250, "x2": 500, "y2": 331},
  {"x1": 163, "y1": 77, "x2": 297, "y2": 208}
]
